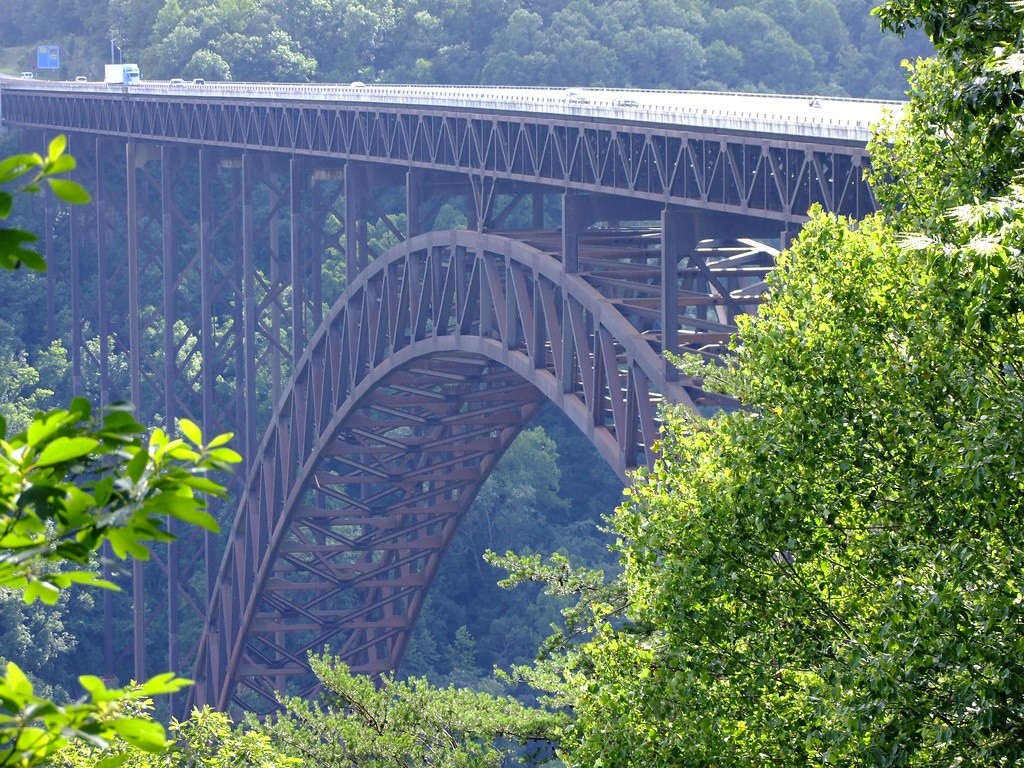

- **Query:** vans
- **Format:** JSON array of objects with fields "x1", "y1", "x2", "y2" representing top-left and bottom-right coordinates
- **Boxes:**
[{"x1": 20, "y1": 72, "x2": 33, "y2": 81}]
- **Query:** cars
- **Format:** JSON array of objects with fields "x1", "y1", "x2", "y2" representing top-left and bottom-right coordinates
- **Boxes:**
[
  {"x1": 561, "y1": 88, "x2": 590, "y2": 104},
  {"x1": 169, "y1": 78, "x2": 186, "y2": 87},
  {"x1": 349, "y1": 81, "x2": 366, "y2": 90},
  {"x1": 76, "y1": 76, "x2": 87, "y2": 83},
  {"x1": 193, "y1": 78, "x2": 204, "y2": 84}
]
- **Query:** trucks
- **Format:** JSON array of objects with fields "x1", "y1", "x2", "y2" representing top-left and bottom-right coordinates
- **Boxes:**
[{"x1": 104, "y1": 63, "x2": 140, "y2": 86}]
